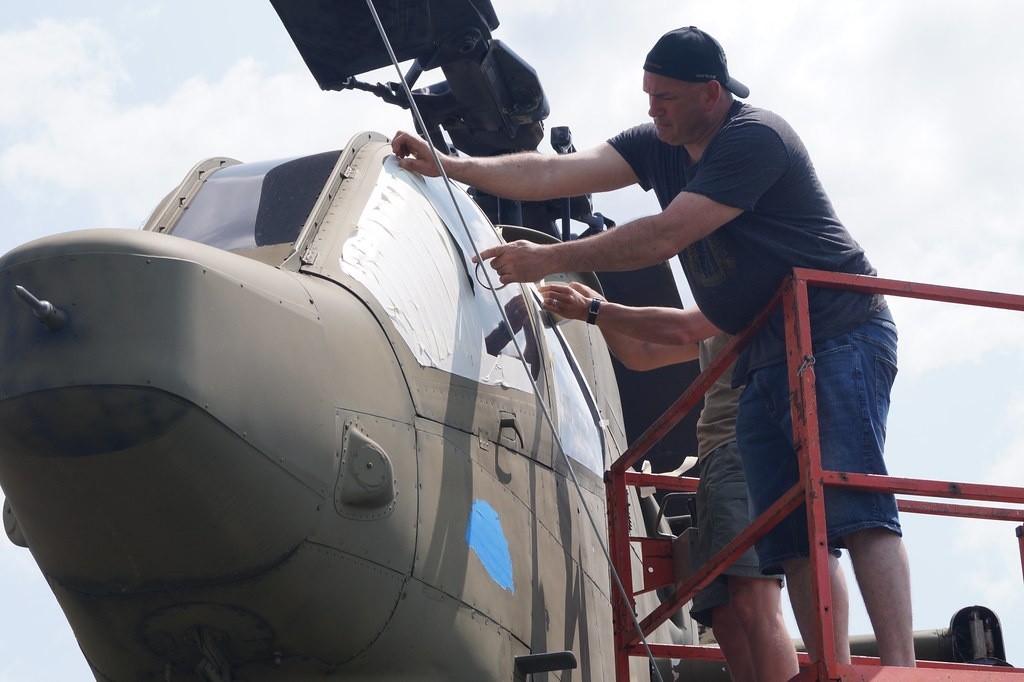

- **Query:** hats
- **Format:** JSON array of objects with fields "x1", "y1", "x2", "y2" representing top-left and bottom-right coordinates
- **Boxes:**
[{"x1": 644, "y1": 26, "x2": 750, "y2": 99}]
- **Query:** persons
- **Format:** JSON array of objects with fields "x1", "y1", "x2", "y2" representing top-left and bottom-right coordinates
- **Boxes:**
[
  {"x1": 393, "y1": 25, "x2": 916, "y2": 666},
  {"x1": 483, "y1": 294, "x2": 540, "y2": 382},
  {"x1": 538, "y1": 281, "x2": 801, "y2": 682}
]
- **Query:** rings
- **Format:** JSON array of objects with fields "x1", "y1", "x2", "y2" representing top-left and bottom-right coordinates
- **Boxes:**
[{"x1": 553, "y1": 299, "x2": 557, "y2": 304}]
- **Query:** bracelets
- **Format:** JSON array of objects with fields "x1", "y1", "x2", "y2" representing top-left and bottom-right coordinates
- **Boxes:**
[
  {"x1": 498, "y1": 320, "x2": 511, "y2": 344},
  {"x1": 587, "y1": 298, "x2": 600, "y2": 325}
]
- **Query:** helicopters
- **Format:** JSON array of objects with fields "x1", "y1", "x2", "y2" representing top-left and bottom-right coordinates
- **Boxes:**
[{"x1": 1, "y1": 1, "x2": 708, "y2": 681}]
